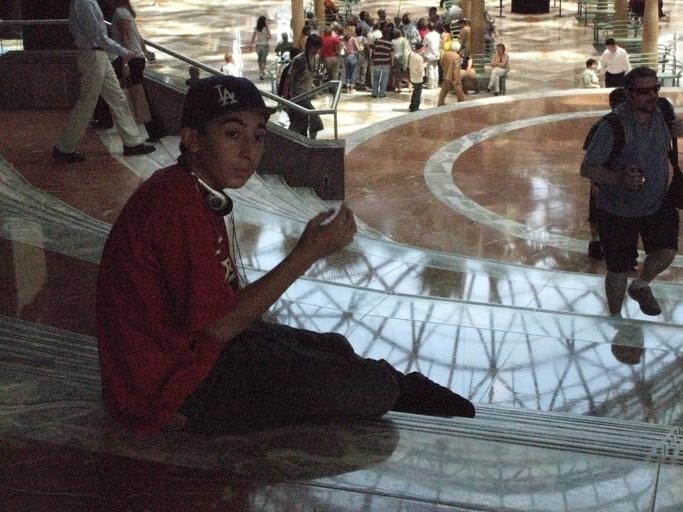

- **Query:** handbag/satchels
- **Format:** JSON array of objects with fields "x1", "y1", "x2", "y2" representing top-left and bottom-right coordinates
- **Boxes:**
[{"x1": 122, "y1": 84, "x2": 152, "y2": 127}]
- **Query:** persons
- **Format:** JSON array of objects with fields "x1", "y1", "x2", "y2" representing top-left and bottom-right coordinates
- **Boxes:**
[
  {"x1": 49, "y1": 0, "x2": 156, "y2": 165},
  {"x1": 274, "y1": 1, "x2": 498, "y2": 112},
  {"x1": 580, "y1": 57, "x2": 601, "y2": 89},
  {"x1": 249, "y1": 16, "x2": 271, "y2": 81},
  {"x1": 219, "y1": 52, "x2": 242, "y2": 78},
  {"x1": 581, "y1": 65, "x2": 682, "y2": 320},
  {"x1": 484, "y1": 43, "x2": 509, "y2": 98},
  {"x1": 101, "y1": 1, "x2": 155, "y2": 137},
  {"x1": 184, "y1": 66, "x2": 202, "y2": 90},
  {"x1": 276, "y1": 34, "x2": 328, "y2": 140},
  {"x1": 592, "y1": 38, "x2": 633, "y2": 88},
  {"x1": 96, "y1": 76, "x2": 475, "y2": 430},
  {"x1": 581, "y1": 89, "x2": 638, "y2": 269},
  {"x1": 609, "y1": 321, "x2": 646, "y2": 365}
]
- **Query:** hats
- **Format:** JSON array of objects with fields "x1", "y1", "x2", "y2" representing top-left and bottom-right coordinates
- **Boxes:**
[{"x1": 181, "y1": 76, "x2": 276, "y2": 130}]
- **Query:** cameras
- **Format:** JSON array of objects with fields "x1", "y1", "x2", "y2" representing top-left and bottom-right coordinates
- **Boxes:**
[
  {"x1": 313, "y1": 63, "x2": 328, "y2": 81},
  {"x1": 633, "y1": 169, "x2": 648, "y2": 189}
]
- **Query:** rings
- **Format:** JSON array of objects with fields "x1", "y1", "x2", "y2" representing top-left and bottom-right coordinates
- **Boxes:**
[
  {"x1": 332, "y1": 219, "x2": 345, "y2": 229},
  {"x1": 641, "y1": 176, "x2": 645, "y2": 185}
]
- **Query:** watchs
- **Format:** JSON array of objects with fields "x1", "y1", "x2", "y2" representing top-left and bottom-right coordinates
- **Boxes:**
[{"x1": 121, "y1": 63, "x2": 129, "y2": 68}]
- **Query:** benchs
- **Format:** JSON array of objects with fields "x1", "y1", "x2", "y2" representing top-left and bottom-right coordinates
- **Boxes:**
[
  {"x1": 575, "y1": 0, "x2": 682, "y2": 87},
  {"x1": 463, "y1": 53, "x2": 508, "y2": 95}
]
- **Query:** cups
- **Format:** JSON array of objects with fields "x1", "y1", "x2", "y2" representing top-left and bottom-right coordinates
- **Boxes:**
[{"x1": 628, "y1": 168, "x2": 647, "y2": 193}]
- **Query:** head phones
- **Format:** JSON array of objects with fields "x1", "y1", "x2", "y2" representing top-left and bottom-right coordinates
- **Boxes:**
[{"x1": 177, "y1": 155, "x2": 233, "y2": 216}]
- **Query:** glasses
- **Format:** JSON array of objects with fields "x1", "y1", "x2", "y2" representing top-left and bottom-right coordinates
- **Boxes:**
[{"x1": 625, "y1": 85, "x2": 660, "y2": 95}]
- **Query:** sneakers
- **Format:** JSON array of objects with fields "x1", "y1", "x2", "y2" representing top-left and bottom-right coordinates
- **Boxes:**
[
  {"x1": 628, "y1": 279, "x2": 662, "y2": 315},
  {"x1": 390, "y1": 371, "x2": 475, "y2": 419},
  {"x1": 144, "y1": 128, "x2": 168, "y2": 142},
  {"x1": 588, "y1": 239, "x2": 604, "y2": 260},
  {"x1": 124, "y1": 143, "x2": 155, "y2": 155},
  {"x1": 52, "y1": 145, "x2": 85, "y2": 162}
]
